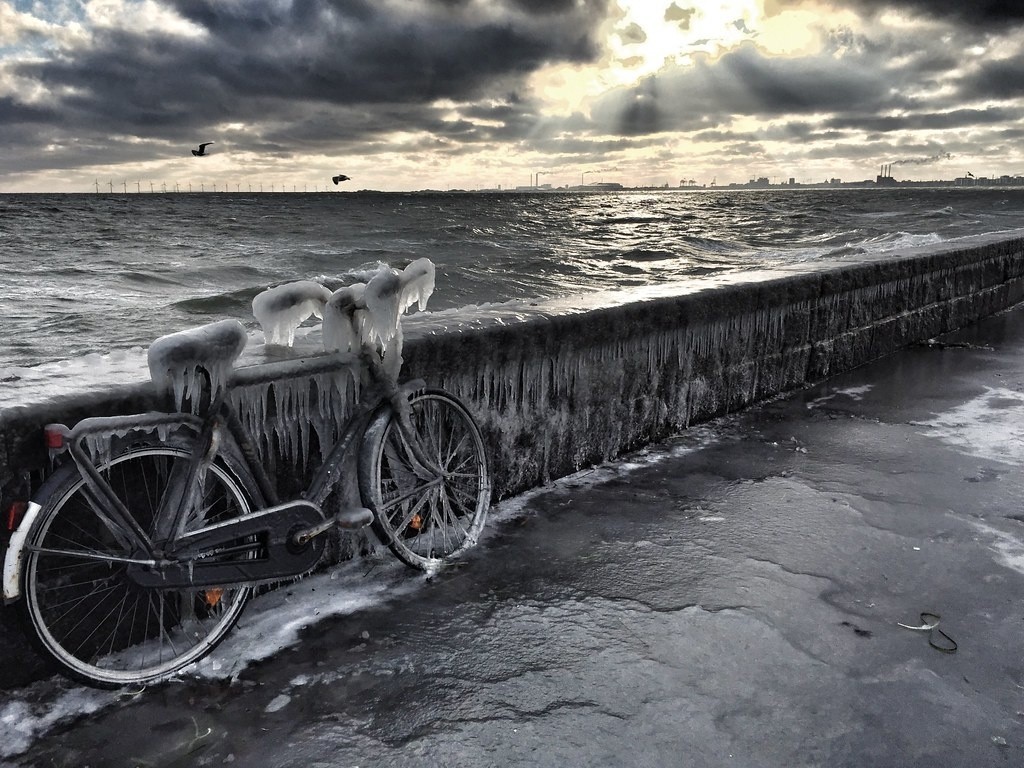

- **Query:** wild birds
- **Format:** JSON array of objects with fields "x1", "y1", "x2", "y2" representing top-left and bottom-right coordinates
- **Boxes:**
[
  {"x1": 191, "y1": 142, "x2": 215, "y2": 157},
  {"x1": 332, "y1": 174, "x2": 351, "y2": 185}
]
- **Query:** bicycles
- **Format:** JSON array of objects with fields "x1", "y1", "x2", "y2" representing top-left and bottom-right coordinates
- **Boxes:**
[{"x1": 0, "y1": 270, "x2": 490, "y2": 691}]
